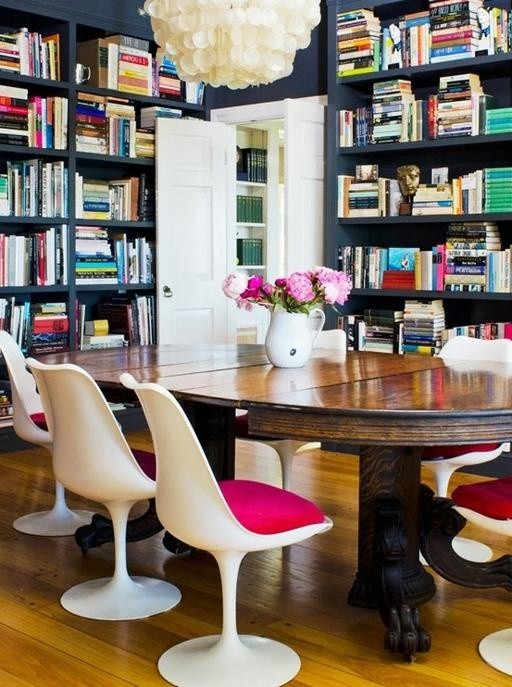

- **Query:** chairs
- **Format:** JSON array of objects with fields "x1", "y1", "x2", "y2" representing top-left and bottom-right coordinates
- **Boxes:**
[
  {"x1": 421, "y1": 336, "x2": 512, "y2": 567},
  {"x1": 23, "y1": 358, "x2": 184, "y2": 621},
  {"x1": 450, "y1": 472, "x2": 512, "y2": 680},
  {"x1": 310, "y1": 328, "x2": 347, "y2": 352},
  {"x1": 120, "y1": 372, "x2": 333, "y2": 687},
  {"x1": 0, "y1": 328, "x2": 123, "y2": 538}
]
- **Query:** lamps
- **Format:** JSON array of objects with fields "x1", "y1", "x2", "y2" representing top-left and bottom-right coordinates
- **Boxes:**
[{"x1": 142, "y1": 0, "x2": 322, "y2": 90}]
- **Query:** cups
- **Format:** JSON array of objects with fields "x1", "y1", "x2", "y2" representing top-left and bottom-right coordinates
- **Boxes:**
[{"x1": 76, "y1": 63, "x2": 91, "y2": 86}]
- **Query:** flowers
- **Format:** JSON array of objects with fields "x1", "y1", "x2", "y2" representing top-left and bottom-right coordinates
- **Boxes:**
[{"x1": 219, "y1": 262, "x2": 358, "y2": 314}]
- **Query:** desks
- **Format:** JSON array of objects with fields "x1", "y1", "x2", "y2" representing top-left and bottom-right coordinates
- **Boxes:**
[{"x1": 31, "y1": 342, "x2": 512, "y2": 663}]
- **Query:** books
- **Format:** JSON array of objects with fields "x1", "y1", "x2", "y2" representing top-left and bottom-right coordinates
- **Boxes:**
[
  {"x1": 335, "y1": 220, "x2": 512, "y2": 293},
  {"x1": 0, "y1": 26, "x2": 62, "y2": 80},
  {"x1": 75, "y1": 30, "x2": 152, "y2": 97},
  {"x1": 0, "y1": 296, "x2": 69, "y2": 355},
  {"x1": 75, "y1": 172, "x2": 156, "y2": 223},
  {"x1": 235, "y1": 145, "x2": 269, "y2": 184},
  {"x1": 0, "y1": 157, "x2": 69, "y2": 222},
  {"x1": 338, "y1": 73, "x2": 510, "y2": 149},
  {"x1": 73, "y1": 226, "x2": 156, "y2": 285},
  {"x1": 77, "y1": 290, "x2": 154, "y2": 352},
  {"x1": 156, "y1": 45, "x2": 205, "y2": 106},
  {"x1": 237, "y1": 194, "x2": 264, "y2": 223},
  {"x1": 336, "y1": 299, "x2": 512, "y2": 357},
  {"x1": 336, "y1": 164, "x2": 512, "y2": 216},
  {"x1": 74, "y1": 88, "x2": 182, "y2": 159},
  {"x1": 0, "y1": 83, "x2": 70, "y2": 151},
  {"x1": 239, "y1": 238, "x2": 263, "y2": 266},
  {"x1": 0, "y1": 222, "x2": 67, "y2": 288},
  {"x1": 1, "y1": 380, "x2": 14, "y2": 418},
  {"x1": 336, "y1": 3, "x2": 512, "y2": 79}
]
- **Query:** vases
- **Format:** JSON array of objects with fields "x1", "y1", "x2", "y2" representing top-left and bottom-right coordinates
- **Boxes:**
[{"x1": 265, "y1": 299, "x2": 326, "y2": 369}]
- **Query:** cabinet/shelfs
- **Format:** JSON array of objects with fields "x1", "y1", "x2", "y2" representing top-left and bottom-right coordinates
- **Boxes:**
[
  {"x1": 236, "y1": 127, "x2": 270, "y2": 282},
  {"x1": 0, "y1": 0, "x2": 210, "y2": 454},
  {"x1": 320, "y1": 0, "x2": 512, "y2": 479}
]
